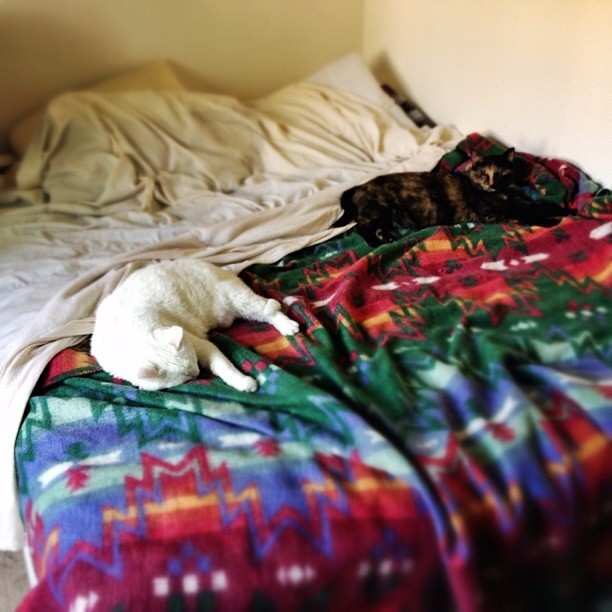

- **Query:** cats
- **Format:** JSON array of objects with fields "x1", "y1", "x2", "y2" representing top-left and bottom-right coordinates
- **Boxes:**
[
  {"x1": 89, "y1": 259, "x2": 299, "y2": 393},
  {"x1": 332, "y1": 147, "x2": 578, "y2": 248}
]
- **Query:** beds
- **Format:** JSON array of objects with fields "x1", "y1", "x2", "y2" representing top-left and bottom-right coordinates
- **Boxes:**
[{"x1": 0, "y1": 55, "x2": 612, "y2": 611}]
